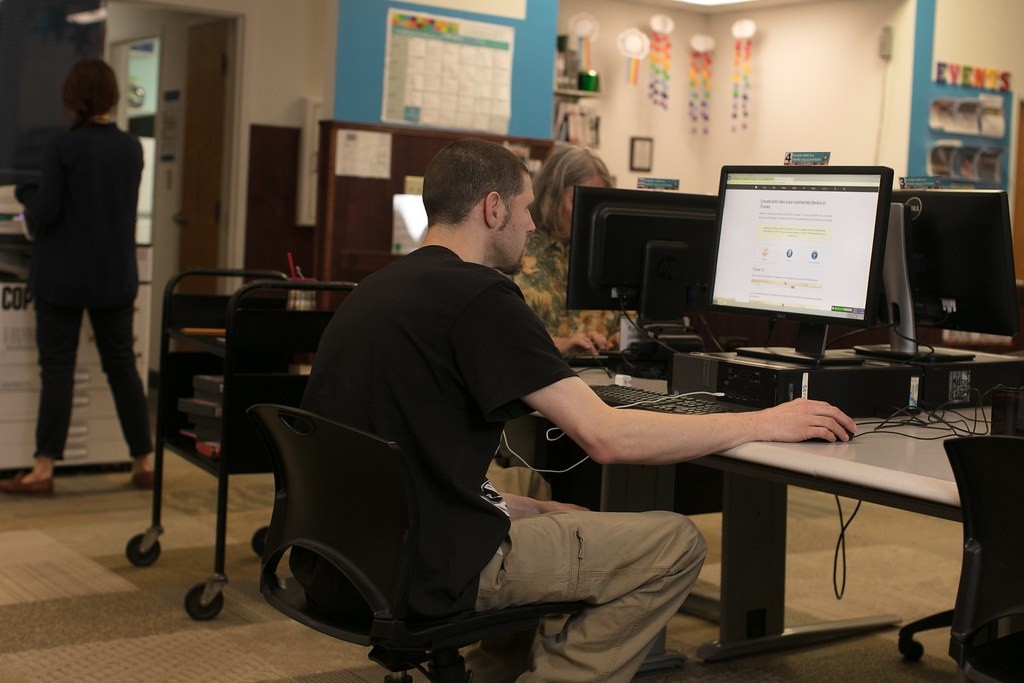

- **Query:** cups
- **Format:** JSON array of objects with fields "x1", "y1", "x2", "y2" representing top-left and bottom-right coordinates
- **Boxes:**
[{"x1": 288, "y1": 277, "x2": 317, "y2": 310}]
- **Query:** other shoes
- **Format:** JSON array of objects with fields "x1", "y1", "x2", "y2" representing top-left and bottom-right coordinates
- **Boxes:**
[
  {"x1": 0, "y1": 472, "x2": 54, "y2": 497},
  {"x1": 129, "y1": 469, "x2": 155, "y2": 490}
]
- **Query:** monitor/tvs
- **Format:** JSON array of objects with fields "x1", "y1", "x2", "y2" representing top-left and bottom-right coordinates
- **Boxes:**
[{"x1": 566, "y1": 165, "x2": 1019, "y2": 366}]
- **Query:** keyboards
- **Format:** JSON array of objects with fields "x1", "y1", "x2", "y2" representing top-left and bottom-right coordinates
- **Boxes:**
[
  {"x1": 572, "y1": 351, "x2": 611, "y2": 368},
  {"x1": 588, "y1": 383, "x2": 734, "y2": 417}
]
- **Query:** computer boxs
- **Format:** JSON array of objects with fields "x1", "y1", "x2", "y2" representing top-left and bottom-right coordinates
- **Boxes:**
[
  {"x1": 669, "y1": 349, "x2": 923, "y2": 418},
  {"x1": 831, "y1": 345, "x2": 1024, "y2": 412}
]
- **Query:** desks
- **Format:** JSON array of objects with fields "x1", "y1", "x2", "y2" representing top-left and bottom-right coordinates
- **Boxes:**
[{"x1": 529, "y1": 343, "x2": 1024, "y2": 674}]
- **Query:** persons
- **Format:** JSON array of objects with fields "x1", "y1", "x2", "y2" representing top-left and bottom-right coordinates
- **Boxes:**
[
  {"x1": 0, "y1": 60, "x2": 155, "y2": 497},
  {"x1": 496, "y1": 146, "x2": 638, "y2": 356},
  {"x1": 302, "y1": 139, "x2": 858, "y2": 683}
]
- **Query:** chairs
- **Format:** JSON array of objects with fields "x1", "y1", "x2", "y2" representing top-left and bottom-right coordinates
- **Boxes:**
[{"x1": 244, "y1": 405, "x2": 585, "y2": 683}]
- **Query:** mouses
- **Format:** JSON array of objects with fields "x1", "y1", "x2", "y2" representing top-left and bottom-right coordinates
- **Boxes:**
[{"x1": 804, "y1": 424, "x2": 854, "y2": 442}]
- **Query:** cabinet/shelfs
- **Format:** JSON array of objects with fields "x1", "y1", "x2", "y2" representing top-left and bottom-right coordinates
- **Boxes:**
[
  {"x1": 553, "y1": 88, "x2": 605, "y2": 148},
  {"x1": 124, "y1": 267, "x2": 358, "y2": 621}
]
